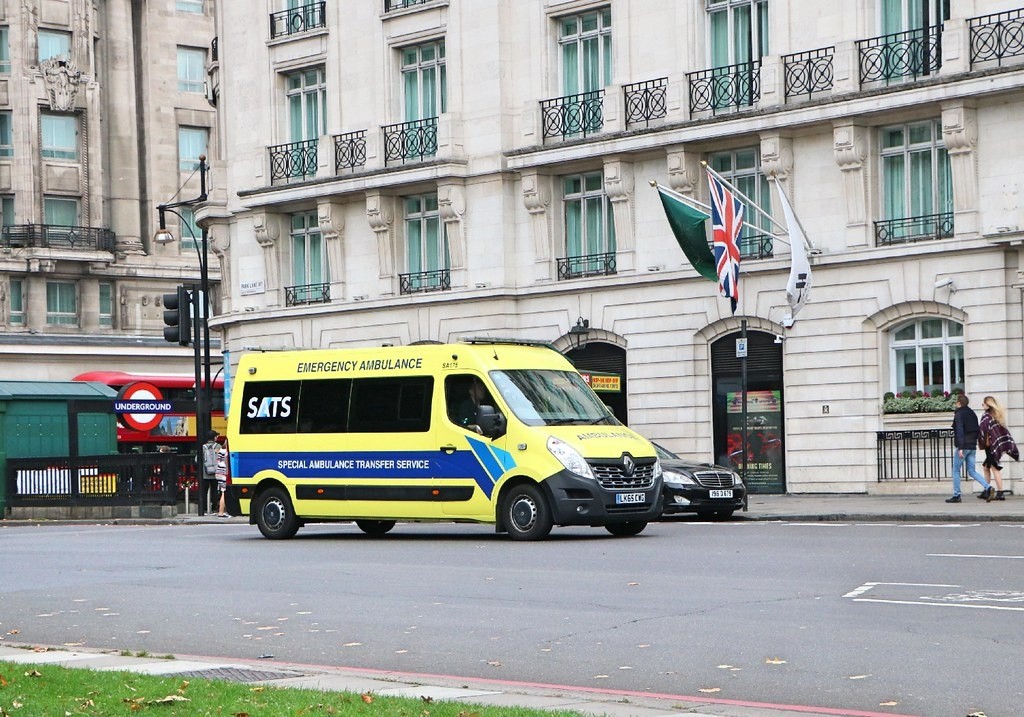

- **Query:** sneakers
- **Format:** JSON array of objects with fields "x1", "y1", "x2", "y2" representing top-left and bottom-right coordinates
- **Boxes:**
[{"x1": 217, "y1": 512, "x2": 232, "y2": 518}]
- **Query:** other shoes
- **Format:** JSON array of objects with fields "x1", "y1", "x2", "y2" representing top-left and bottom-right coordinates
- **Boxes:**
[
  {"x1": 945, "y1": 495, "x2": 961, "y2": 503},
  {"x1": 986, "y1": 487, "x2": 995, "y2": 502},
  {"x1": 992, "y1": 491, "x2": 1005, "y2": 500},
  {"x1": 976, "y1": 490, "x2": 985, "y2": 499}
]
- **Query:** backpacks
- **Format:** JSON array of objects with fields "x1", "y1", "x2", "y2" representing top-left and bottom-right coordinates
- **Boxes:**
[{"x1": 203, "y1": 442, "x2": 218, "y2": 475}]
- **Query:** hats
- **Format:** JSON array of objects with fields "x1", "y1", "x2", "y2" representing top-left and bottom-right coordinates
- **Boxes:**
[
  {"x1": 206, "y1": 430, "x2": 220, "y2": 440},
  {"x1": 216, "y1": 436, "x2": 227, "y2": 444}
]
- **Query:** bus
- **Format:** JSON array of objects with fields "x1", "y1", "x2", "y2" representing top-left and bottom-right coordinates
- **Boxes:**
[{"x1": 72, "y1": 371, "x2": 224, "y2": 493}]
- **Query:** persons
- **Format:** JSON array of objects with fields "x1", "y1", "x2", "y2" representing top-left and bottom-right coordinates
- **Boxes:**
[
  {"x1": 458, "y1": 379, "x2": 488, "y2": 429},
  {"x1": 976, "y1": 396, "x2": 1019, "y2": 501},
  {"x1": 945, "y1": 394, "x2": 995, "y2": 503},
  {"x1": 154, "y1": 430, "x2": 232, "y2": 519}
]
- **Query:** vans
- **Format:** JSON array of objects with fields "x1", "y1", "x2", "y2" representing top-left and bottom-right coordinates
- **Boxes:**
[{"x1": 227, "y1": 337, "x2": 664, "y2": 541}]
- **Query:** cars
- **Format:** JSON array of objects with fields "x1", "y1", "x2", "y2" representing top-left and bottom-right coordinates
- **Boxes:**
[{"x1": 650, "y1": 441, "x2": 746, "y2": 521}]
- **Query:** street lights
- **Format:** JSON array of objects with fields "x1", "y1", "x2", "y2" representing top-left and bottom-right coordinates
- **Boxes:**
[{"x1": 152, "y1": 204, "x2": 211, "y2": 440}]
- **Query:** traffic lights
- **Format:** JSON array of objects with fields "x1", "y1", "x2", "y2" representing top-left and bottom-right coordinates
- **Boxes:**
[{"x1": 163, "y1": 286, "x2": 191, "y2": 347}]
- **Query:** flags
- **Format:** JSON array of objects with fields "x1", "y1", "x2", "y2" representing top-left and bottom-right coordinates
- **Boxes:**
[
  {"x1": 774, "y1": 178, "x2": 812, "y2": 319},
  {"x1": 659, "y1": 190, "x2": 718, "y2": 283},
  {"x1": 707, "y1": 172, "x2": 745, "y2": 318}
]
